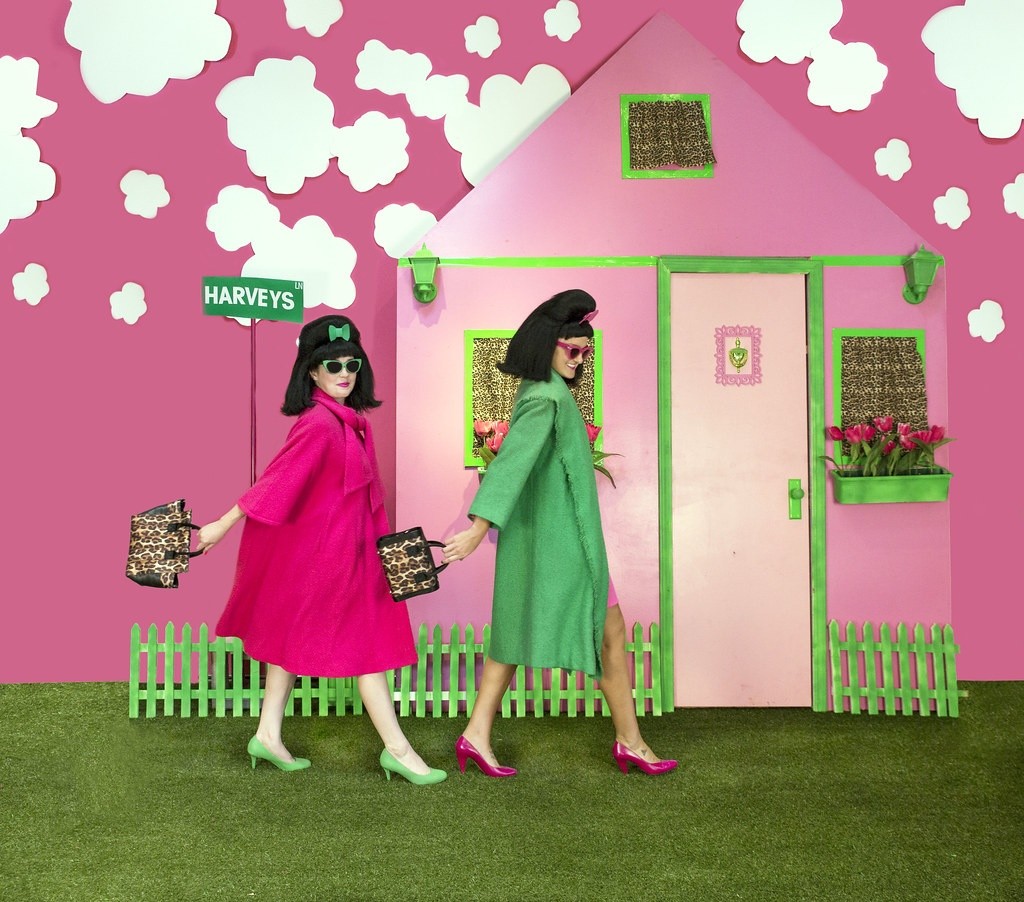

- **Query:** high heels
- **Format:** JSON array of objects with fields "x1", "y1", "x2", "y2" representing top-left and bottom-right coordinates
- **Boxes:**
[
  {"x1": 247, "y1": 735, "x2": 311, "y2": 771},
  {"x1": 613, "y1": 740, "x2": 678, "y2": 775},
  {"x1": 380, "y1": 748, "x2": 447, "y2": 785},
  {"x1": 455, "y1": 736, "x2": 517, "y2": 777}
]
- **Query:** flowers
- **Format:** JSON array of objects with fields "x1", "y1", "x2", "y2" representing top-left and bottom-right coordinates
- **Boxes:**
[
  {"x1": 584, "y1": 421, "x2": 620, "y2": 487},
  {"x1": 818, "y1": 416, "x2": 954, "y2": 475},
  {"x1": 473, "y1": 418, "x2": 510, "y2": 468}
]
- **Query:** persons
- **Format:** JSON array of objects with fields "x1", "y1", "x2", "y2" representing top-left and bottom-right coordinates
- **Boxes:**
[
  {"x1": 439, "y1": 289, "x2": 681, "y2": 776},
  {"x1": 198, "y1": 309, "x2": 447, "y2": 785}
]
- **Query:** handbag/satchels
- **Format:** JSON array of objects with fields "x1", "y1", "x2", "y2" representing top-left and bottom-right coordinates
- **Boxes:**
[
  {"x1": 125, "y1": 499, "x2": 205, "y2": 589},
  {"x1": 376, "y1": 526, "x2": 450, "y2": 602}
]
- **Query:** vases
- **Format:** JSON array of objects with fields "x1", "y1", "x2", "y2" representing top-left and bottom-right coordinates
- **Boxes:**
[{"x1": 829, "y1": 466, "x2": 953, "y2": 503}]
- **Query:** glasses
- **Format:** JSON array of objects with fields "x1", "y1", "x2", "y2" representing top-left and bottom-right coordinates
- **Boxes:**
[
  {"x1": 557, "y1": 341, "x2": 594, "y2": 359},
  {"x1": 320, "y1": 359, "x2": 362, "y2": 374}
]
